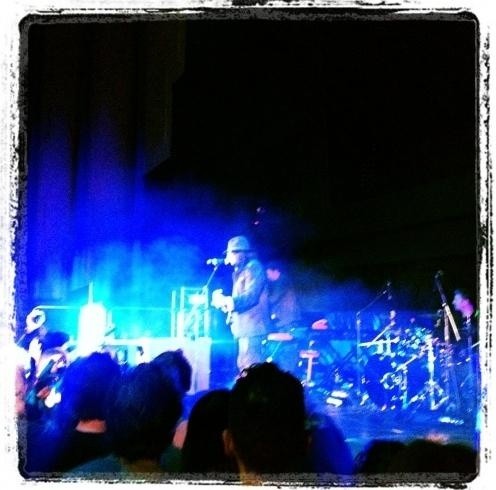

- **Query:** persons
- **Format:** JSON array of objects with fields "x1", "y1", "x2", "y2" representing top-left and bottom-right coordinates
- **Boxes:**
[
  {"x1": 14, "y1": 308, "x2": 480, "y2": 483},
  {"x1": 210, "y1": 234, "x2": 272, "y2": 378},
  {"x1": 451, "y1": 290, "x2": 479, "y2": 431},
  {"x1": 264, "y1": 260, "x2": 305, "y2": 376}
]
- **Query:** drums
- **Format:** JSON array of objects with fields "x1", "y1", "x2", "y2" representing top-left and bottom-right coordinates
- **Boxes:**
[{"x1": 365, "y1": 354, "x2": 430, "y2": 410}]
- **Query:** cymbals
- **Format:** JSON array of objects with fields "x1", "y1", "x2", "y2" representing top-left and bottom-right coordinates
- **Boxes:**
[{"x1": 419, "y1": 314, "x2": 445, "y2": 322}]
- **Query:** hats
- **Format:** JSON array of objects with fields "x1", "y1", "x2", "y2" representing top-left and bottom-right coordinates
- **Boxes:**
[{"x1": 225, "y1": 236, "x2": 255, "y2": 255}]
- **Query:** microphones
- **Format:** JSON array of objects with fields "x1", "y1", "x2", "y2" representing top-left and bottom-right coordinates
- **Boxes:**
[{"x1": 206, "y1": 258, "x2": 230, "y2": 266}]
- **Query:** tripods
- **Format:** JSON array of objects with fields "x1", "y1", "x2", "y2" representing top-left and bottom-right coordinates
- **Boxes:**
[{"x1": 339, "y1": 289, "x2": 481, "y2": 427}]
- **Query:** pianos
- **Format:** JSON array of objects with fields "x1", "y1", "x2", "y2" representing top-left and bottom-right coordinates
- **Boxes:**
[{"x1": 290, "y1": 327, "x2": 396, "y2": 340}]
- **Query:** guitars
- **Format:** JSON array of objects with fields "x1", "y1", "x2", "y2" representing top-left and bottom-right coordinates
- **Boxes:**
[{"x1": 211, "y1": 289, "x2": 233, "y2": 326}]
